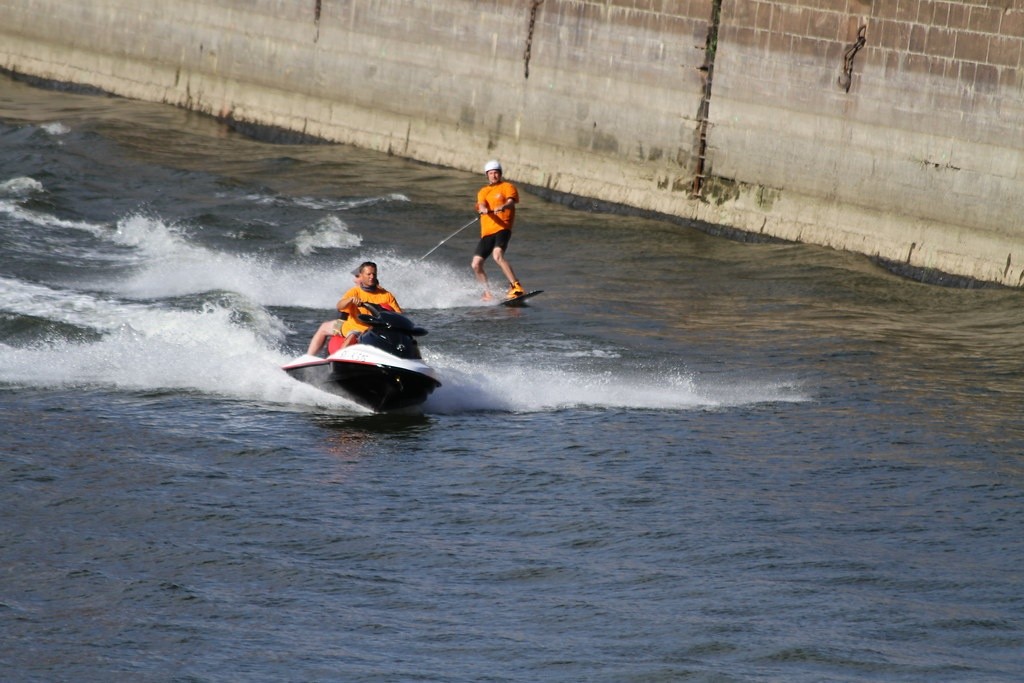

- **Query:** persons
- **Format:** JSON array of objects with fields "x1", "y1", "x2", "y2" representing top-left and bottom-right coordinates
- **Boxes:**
[
  {"x1": 307, "y1": 262, "x2": 403, "y2": 356},
  {"x1": 472, "y1": 160, "x2": 524, "y2": 302}
]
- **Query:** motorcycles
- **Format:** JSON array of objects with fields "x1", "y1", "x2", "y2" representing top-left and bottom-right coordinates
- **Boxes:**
[{"x1": 282, "y1": 300, "x2": 443, "y2": 416}]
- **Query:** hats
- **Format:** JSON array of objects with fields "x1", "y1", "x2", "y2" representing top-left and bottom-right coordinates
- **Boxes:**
[{"x1": 350, "y1": 267, "x2": 360, "y2": 275}]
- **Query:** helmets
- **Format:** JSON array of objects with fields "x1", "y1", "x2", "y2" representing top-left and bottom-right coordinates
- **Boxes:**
[{"x1": 485, "y1": 160, "x2": 501, "y2": 174}]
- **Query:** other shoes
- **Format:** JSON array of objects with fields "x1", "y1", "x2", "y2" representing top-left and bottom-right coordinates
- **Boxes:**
[{"x1": 506, "y1": 281, "x2": 526, "y2": 300}]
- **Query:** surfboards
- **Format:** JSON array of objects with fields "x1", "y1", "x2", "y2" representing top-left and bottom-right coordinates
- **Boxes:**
[{"x1": 497, "y1": 289, "x2": 545, "y2": 304}]
